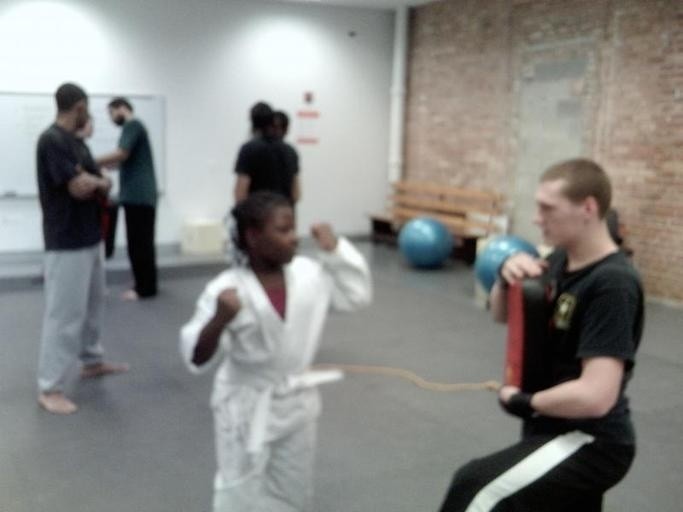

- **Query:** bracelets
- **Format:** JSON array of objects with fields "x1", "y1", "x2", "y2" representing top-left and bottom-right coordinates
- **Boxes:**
[{"x1": 494, "y1": 252, "x2": 508, "y2": 290}]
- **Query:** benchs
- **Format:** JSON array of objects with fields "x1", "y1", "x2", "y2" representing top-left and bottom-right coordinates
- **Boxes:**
[{"x1": 371, "y1": 182, "x2": 506, "y2": 266}]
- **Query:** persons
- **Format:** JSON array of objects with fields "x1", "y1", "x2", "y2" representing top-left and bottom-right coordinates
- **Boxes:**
[
  {"x1": 180, "y1": 189, "x2": 375, "y2": 512},
  {"x1": 91, "y1": 96, "x2": 159, "y2": 300},
  {"x1": 232, "y1": 99, "x2": 302, "y2": 205},
  {"x1": 274, "y1": 112, "x2": 289, "y2": 140},
  {"x1": 35, "y1": 84, "x2": 129, "y2": 413},
  {"x1": 437, "y1": 157, "x2": 646, "y2": 510}
]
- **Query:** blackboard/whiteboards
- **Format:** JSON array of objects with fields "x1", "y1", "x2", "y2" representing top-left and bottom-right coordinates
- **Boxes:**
[{"x1": 0, "y1": 90, "x2": 164, "y2": 198}]
